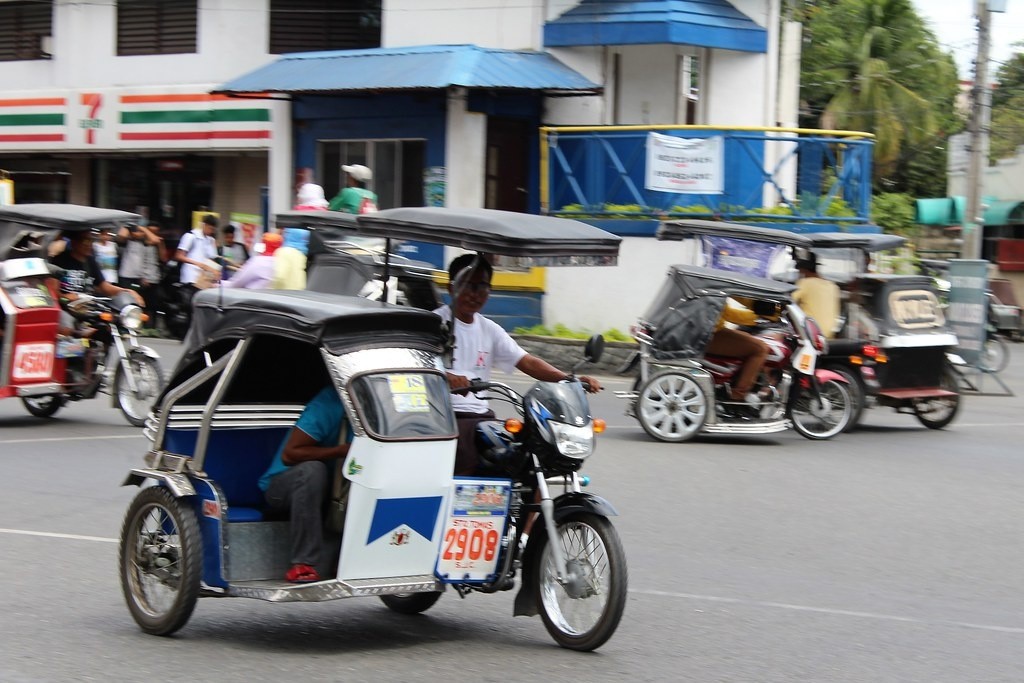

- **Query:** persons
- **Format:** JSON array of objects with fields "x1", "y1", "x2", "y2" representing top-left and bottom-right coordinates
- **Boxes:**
[
  {"x1": 173, "y1": 214, "x2": 220, "y2": 338},
  {"x1": 216, "y1": 225, "x2": 248, "y2": 279},
  {"x1": 329, "y1": 163, "x2": 379, "y2": 216},
  {"x1": 279, "y1": 184, "x2": 330, "y2": 254},
  {"x1": 705, "y1": 292, "x2": 783, "y2": 416},
  {"x1": 245, "y1": 374, "x2": 380, "y2": 583},
  {"x1": 213, "y1": 233, "x2": 284, "y2": 289},
  {"x1": 47, "y1": 222, "x2": 169, "y2": 380},
  {"x1": 779, "y1": 253, "x2": 840, "y2": 338},
  {"x1": 427, "y1": 254, "x2": 600, "y2": 552}
]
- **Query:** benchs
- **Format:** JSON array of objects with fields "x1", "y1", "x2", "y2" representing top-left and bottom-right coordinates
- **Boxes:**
[{"x1": 164, "y1": 426, "x2": 332, "y2": 582}]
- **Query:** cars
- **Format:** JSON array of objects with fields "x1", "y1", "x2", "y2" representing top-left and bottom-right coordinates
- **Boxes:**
[{"x1": 984, "y1": 289, "x2": 1024, "y2": 342}]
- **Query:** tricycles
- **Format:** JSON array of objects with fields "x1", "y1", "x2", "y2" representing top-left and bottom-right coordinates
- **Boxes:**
[
  {"x1": 613, "y1": 219, "x2": 1009, "y2": 443},
  {"x1": 117, "y1": 207, "x2": 627, "y2": 652},
  {"x1": 0, "y1": 204, "x2": 166, "y2": 428}
]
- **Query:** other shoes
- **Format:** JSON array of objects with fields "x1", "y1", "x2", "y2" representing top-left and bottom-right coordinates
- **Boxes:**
[
  {"x1": 724, "y1": 398, "x2": 760, "y2": 419},
  {"x1": 284, "y1": 564, "x2": 319, "y2": 583}
]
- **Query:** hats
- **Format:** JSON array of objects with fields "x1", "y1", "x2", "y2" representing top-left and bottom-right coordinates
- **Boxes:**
[
  {"x1": 341, "y1": 163, "x2": 373, "y2": 183},
  {"x1": 794, "y1": 252, "x2": 821, "y2": 269}
]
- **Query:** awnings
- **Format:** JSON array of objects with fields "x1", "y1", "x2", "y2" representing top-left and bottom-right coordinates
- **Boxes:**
[{"x1": 206, "y1": 46, "x2": 604, "y2": 106}]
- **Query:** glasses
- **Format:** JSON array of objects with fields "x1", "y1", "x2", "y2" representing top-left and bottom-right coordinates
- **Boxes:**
[{"x1": 458, "y1": 279, "x2": 492, "y2": 291}]
